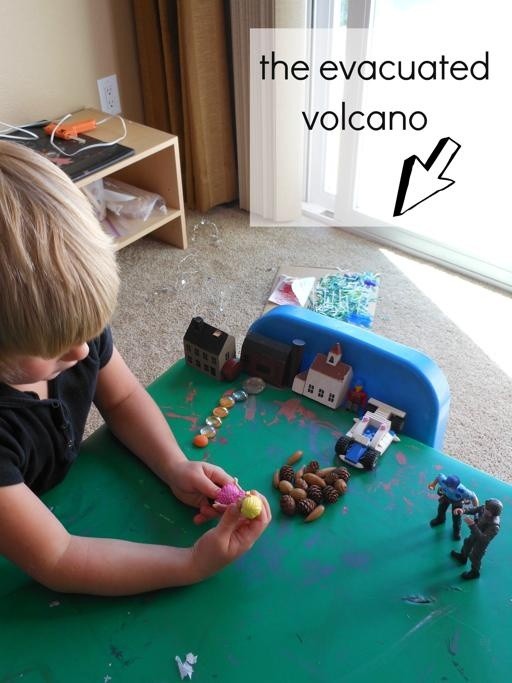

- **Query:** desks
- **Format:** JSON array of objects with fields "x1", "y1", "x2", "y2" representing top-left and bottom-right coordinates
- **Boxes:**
[{"x1": 0, "y1": 356, "x2": 512, "y2": 683}]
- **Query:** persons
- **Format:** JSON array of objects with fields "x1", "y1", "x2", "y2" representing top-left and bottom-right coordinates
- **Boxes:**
[{"x1": 0, "y1": 132, "x2": 272, "y2": 599}]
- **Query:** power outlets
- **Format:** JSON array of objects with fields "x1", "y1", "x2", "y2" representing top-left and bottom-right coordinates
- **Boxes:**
[{"x1": 96, "y1": 74, "x2": 122, "y2": 115}]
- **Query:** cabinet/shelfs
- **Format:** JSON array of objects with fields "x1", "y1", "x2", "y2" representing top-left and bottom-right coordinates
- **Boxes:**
[{"x1": 49, "y1": 107, "x2": 189, "y2": 254}]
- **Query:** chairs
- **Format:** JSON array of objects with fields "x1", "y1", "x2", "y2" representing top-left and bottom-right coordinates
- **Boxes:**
[{"x1": 239, "y1": 304, "x2": 452, "y2": 451}]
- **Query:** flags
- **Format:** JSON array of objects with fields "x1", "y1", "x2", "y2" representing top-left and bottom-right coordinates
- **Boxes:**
[
  {"x1": 290, "y1": 340, "x2": 354, "y2": 411},
  {"x1": 450, "y1": 498, "x2": 503, "y2": 580},
  {"x1": 343, "y1": 378, "x2": 369, "y2": 412},
  {"x1": 332, "y1": 396, "x2": 407, "y2": 472},
  {"x1": 206, "y1": 476, "x2": 262, "y2": 521},
  {"x1": 427, "y1": 472, "x2": 479, "y2": 539},
  {"x1": 182, "y1": 316, "x2": 238, "y2": 381},
  {"x1": 241, "y1": 330, "x2": 307, "y2": 392}
]
